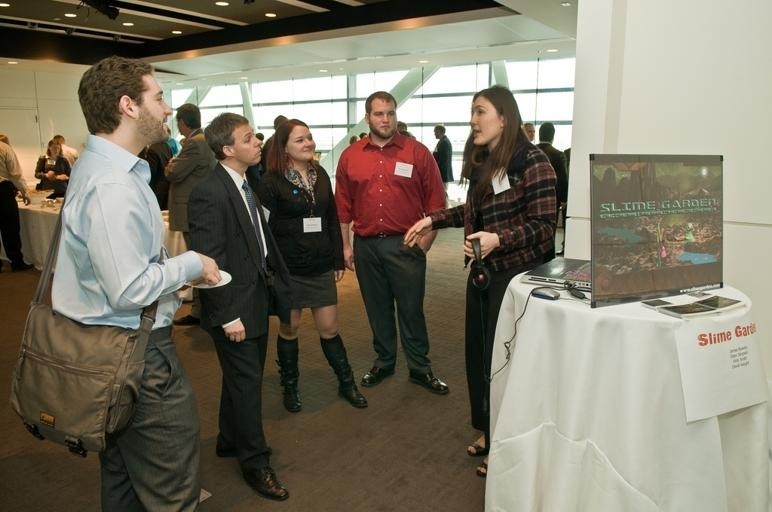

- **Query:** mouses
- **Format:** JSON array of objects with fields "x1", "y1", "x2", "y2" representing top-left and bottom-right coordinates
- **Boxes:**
[{"x1": 532, "y1": 287, "x2": 560, "y2": 300}]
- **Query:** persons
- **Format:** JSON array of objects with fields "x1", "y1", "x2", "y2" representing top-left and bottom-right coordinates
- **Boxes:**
[
  {"x1": 402, "y1": 84, "x2": 560, "y2": 479},
  {"x1": 256, "y1": 132, "x2": 264, "y2": 141},
  {"x1": 32, "y1": 139, "x2": 74, "y2": 199},
  {"x1": 433, "y1": 125, "x2": 454, "y2": 208},
  {"x1": 48, "y1": 51, "x2": 226, "y2": 510},
  {"x1": 141, "y1": 141, "x2": 173, "y2": 211},
  {"x1": 253, "y1": 114, "x2": 371, "y2": 414},
  {"x1": 556, "y1": 148, "x2": 570, "y2": 255},
  {"x1": 360, "y1": 132, "x2": 367, "y2": 138},
  {"x1": 398, "y1": 121, "x2": 417, "y2": 139},
  {"x1": 350, "y1": 135, "x2": 361, "y2": 145},
  {"x1": 273, "y1": 114, "x2": 288, "y2": 129},
  {"x1": 524, "y1": 123, "x2": 535, "y2": 141},
  {"x1": 165, "y1": 103, "x2": 217, "y2": 303},
  {"x1": 186, "y1": 112, "x2": 296, "y2": 501},
  {"x1": 330, "y1": 91, "x2": 452, "y2": 400},
  {"x1": 535, "y1": 122, "x2": 567, "y2": 237},
  {"x1": 54, "y1": 135, "x2": 81, "y2": 170},
  {"x1": 1, "y1": 132, "x2": 37, "y2": 274},
  {"x1": 163, "y1": 125, "x2": 178, "y2": 154}
]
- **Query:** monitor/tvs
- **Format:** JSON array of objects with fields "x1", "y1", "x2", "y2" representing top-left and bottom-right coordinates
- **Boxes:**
[{"x1": 590, "y1": 154, "x2": 724, "y2": 307}]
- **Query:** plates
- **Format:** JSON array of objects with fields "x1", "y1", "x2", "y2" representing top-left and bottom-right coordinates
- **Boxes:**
[
  {"x1": 185, "y1": 269, "x2": 234, "y2": 290},
  {"x1": 57, "y1": 176, "x2": 70, "y2": 180}
]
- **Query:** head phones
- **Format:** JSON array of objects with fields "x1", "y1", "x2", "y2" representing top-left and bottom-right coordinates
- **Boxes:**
[{"x1": 470, "y1": 238, "x2": 490, "y2": 290}]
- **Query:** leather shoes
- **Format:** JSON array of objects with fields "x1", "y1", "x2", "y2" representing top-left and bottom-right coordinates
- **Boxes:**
[
  {"x1": 408, "y1": 372, "x2": 449, "y2": 394},
  {"x1": 11, "y1": 262, "x2": 33, "y2": 273},
  {"x1": 173, "y1": 315, "x2": 201, "y2": 326},
  {"x1": 360, "y1": 365, "x2": 395, "y2": 386},
  {"x1": 215, "y1": 441, "x2": 271, "y2": 458},
  {"x1": 242, "y1": 467, "x2": 289, "y2": 500}
]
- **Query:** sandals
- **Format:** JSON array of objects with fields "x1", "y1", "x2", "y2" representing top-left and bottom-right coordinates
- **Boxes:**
[
  {"x1": 467, "y1": 440, "x2": 485, "y2": 456},
  {"x1": 476, "y1": 461, "x2": 487, "y2": 477}
]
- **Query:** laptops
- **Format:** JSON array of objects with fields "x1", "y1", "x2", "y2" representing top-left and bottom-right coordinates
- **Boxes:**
[{"x1": 522, "y1": 257, "x2": 591, "y2": 290}]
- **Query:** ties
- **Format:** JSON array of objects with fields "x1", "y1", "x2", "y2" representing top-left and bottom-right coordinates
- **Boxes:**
[{"x1": 242, "y1": 179, "x2": 268, "y2": 273}]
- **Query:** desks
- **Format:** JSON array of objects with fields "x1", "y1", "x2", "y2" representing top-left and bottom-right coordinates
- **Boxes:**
[
  {"x1": 2, "y1": 190, "x2": 203, "y2": 323},
  {"x1": 482, "y1": 260, "x2": 772, "y2": 512}
]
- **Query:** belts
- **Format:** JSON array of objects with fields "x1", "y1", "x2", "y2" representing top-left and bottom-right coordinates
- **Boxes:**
[{"x1": 368, "y1": 232, "x2": 401, "y2": 238}]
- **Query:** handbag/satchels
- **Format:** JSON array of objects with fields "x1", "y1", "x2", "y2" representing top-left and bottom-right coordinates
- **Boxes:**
[{"x1": 8, "y1": 302, "x2": 146, "y2": 458}]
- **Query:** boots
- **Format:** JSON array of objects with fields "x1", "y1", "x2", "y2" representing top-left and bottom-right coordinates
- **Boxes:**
[
  {"x1": 320, "y1": 334, "x2": 367, "y2": 408},
  {"x1": 275, "y1": 335, "x2": 302, "y2": 412}
]
